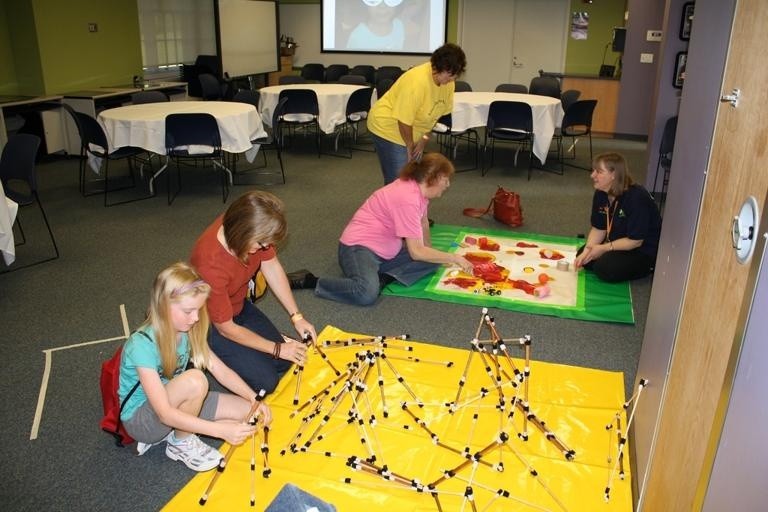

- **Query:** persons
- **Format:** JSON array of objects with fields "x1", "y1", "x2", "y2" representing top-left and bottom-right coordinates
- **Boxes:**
[
  {"x1": 117, "y1": 262, "x2": 272, "y2": 472},
  {"x1": 188, "y1": 190, "x2": 317, "y2": 394},
  {"x1": 287, "y1": 152, "x2": 475, "y2": 306},
  {"x1": 367, "y1": 42, "x2": 466, "y2": 186},
  {"x1": 574, "y1": 152, "x2": 662, "y2": 283}
]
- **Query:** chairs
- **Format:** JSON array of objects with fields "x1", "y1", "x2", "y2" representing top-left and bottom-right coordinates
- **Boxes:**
[
  {"x1": 455, "y1": 80, "x2": 480, "y2": 140},
  {"x1": 0, "y1": 133, "x2": 59, "y2": 274},
  {"x1": 552, "y1": 99, "x2": 598, "y2": 177},
  {"x1": 376, "y1": 66, "x2": 400, "y2": 83},
  {"x1": 557, "y1": 89, "x2": 581, "y2": 160},
  {"x1": 326, "y1": 86, "x2": 377, "y2": 159},
  {"x1": 62, "y1": 103, "x2": 157, "y2": 208},
  {"x1": 197, "y1": 73, "x2": 222, "y2": 102},
  {"x1": 325, "y1": 64, "x2": 349, "y2": 82},
  {"x1": 351, "y1": 65, "x2": 376, "y2": 84},
  {"x1": 494, "y1": 83, "x2": 528, "y2": 94},
  {"x1": 337, "y1": 75, "x2": 368, "y2": 84},
  {"x1": 300, "y1": 63, "x2": 324, "y2": 82},
  {"x1": 528, "y1": 76, "x2": 560, "y2": 99},
  {"x1": 481, "y1": 100, "x2": 534, "y2": 182},
  {"x1": 431, "y1": 113, "x2": 478, "y2": 173},
  {"x1": 231, "y1": 89, "x2": 267, "y2": 184},
  {"x1": 279, "y1": 75, "x2": 304, "y2": 84},
  {"x1": 164, "y1": 113, "x2": 229, "y2": 205},
  {"x1": 275, "y1": 88, "x2": 324, "y2": 159}
]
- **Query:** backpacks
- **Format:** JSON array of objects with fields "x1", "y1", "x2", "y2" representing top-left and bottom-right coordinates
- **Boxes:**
[{"x1": 100, "y1": 330, "x2": 154, "y2": 448}]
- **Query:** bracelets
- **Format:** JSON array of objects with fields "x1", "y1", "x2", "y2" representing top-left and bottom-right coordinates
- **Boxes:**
[
  {"x1": 422, "y1": 134, "x2": 432, "y2": 140},
  {"x1": 290, "y1": 312, "x2": 302, "y2": 317},
  {"x1": 291, "y1": 315, "x2": 304, "y2": 325},
  {"x1": 272, "y1": 341, "x2": 281, "y2": 360},
  {"x1": 609, "y1": 240, "x2": 614, "y2": 251}
]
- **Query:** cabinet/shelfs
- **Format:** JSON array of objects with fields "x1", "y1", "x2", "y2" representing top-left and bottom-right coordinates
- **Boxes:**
[
  {"x1": 56, "y1": 79, "x2": 188, "y2": 160},
  {"x1": 634, "y1": 0, "x2": 768, "y2": 512},
  {"x1": 0, "y1": 91, "x2": 63, "y2": 164}
]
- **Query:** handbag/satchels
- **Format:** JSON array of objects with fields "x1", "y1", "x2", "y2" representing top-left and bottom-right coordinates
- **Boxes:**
[{"x1": 463, "y1": 184, "x2": 524, "y2": 228}]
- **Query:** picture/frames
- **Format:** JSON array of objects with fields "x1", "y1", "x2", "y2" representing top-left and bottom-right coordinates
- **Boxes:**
[
  {"x1": 672, "y1": 51, "x2": 687, "y2": 89},
  {"x1": 679, "y1": 2, "x2": 694, "y2": 41}
]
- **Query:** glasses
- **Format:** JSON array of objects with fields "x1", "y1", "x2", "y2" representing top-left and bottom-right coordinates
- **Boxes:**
[{"x1": 256, "y1": 239, "x2": 272, "y2": 252}]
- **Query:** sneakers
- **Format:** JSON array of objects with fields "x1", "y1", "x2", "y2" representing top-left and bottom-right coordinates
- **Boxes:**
[
  {"x1": 133, "y1": 432, "x2": 173, "y2": 458},
  {"x1": 286, "y1": 269, "x2": 313, "y2": 290},
  {"x1": 164, "y1": 434, "x2": 224, "y2": 473}
]
